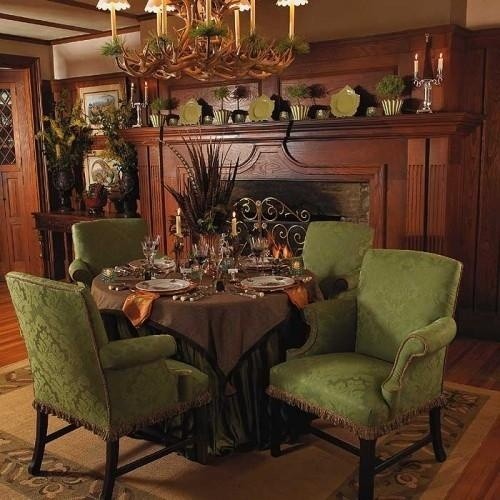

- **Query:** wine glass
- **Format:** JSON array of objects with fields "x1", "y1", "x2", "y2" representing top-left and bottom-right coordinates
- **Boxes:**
[
  {"x1": 191, "y1": 242, "x2": 237, "y2": 294},
  {"x1": 140, "y1": 235, "x2": 159, "y2": 270},
  {"x1": 249, "y1": 231, "x2": 269, "y2": 276}
]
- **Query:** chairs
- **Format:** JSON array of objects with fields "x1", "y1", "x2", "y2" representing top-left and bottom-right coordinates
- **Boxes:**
[
  {"x1": 287, "y1": 220, "x2": 374, "y2": 347},
  {"x1": 265, "y1": 249, "x2": 463, "y2": 500},
  {"x1": 68, "y1": 218, "x2": 171, "y2": 341},
  {"x1": 5, "y1": 271, "x2": 212, "y2": 500}
]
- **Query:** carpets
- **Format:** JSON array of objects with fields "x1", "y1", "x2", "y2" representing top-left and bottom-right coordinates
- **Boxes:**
[{"x1": 0, "y1": 358, "x2": 500, "y2": 500}]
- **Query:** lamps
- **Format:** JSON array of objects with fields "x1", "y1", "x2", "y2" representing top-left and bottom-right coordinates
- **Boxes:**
[{"x1": 96, "y1": 0, "x2": 309, "y2": 82}]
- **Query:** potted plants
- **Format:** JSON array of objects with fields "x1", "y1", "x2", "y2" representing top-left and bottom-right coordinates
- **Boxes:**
[
  {"x1": 34, "y1": 87, "x2": 81, "y2": 212},
  {"x1": 104, "y1": 109, "x2": 140, "y2": 216},
  {"x1": 376, "y1": 74, "x2": 406, "y2": 116},
  {"x1": 212, "y1": 85, "x2": 231, "y2": 124},
  {"x1": 286, "y1": 83, "x2": 310, "y2": 120},
  {"x1": 149, "y1": 98, "x2": 169, "y2": 127},
  {"x1": 83, "y1": 183, "x2": 108, "y2": 214}
]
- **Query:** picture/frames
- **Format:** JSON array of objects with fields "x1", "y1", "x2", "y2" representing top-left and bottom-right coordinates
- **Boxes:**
[
  {"x1": 80, "y1": 149, "x2": 123, "y2": 199},
  {"x1": 80, "y1": 84, "x2": 123, "y2": 135}
]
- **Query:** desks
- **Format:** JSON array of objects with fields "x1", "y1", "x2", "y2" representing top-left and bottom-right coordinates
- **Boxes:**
[{"x1": 31, "y1": 210, "x2": 127, "y2": 282}]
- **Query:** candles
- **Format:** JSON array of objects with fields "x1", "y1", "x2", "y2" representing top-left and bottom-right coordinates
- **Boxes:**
[
  {"x1": 131, "y1": 83, "x2": 134, "y2": 97},
  {"x1": 145, "y1": 81, "x2": 148, "y2": 100},
  {"x1": 231, "y1": 211, "x2": 237, "y2": 234},
  {"x1": 176, "y1": 208, "x2": 182, "y2": 234},
  {"x1": 438, "y1": 53, "x2": 444, "y2": 70},
  {"x1": 414, "y1": 53, "x2": 419, "y2": 72}
]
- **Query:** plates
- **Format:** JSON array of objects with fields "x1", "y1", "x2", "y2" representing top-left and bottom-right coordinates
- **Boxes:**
[
  {"x1": 130, "y1": 259, "x2": 176, "y2": 270},
  {"x1": 249, "y1": 95, "x2": 275, "y2": 121},
  {"x1": 246, "y1": 256, "x2": 277, "y2": 268},
  {"x1": 136, "y1": 278, "x2": 191, "y2": 292},
  {"x1": 179, "y1": 99, "x2": 202, "y2": 125},
  {"x1": 240, "y1": 275, "x2": 294, "y2": 288},
  {"x1": 331, "y1": 85, "x2": 361, "y2": 118}
]
